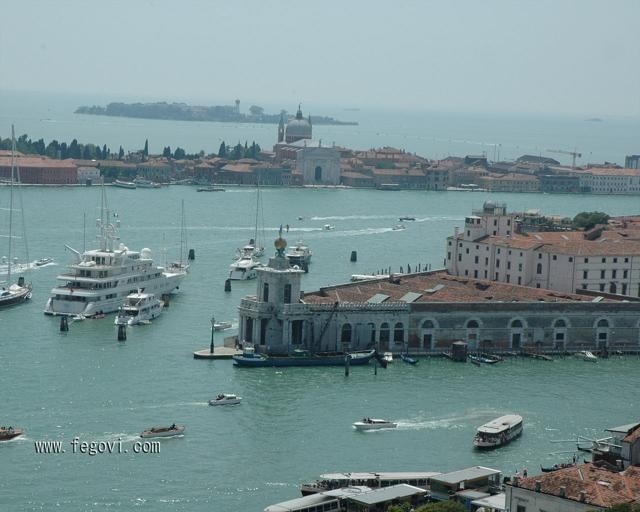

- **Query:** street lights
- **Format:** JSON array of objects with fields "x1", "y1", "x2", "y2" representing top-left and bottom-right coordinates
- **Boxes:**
[{"x1": 209, "y1": 318, "x2": 216, "y2": 352}]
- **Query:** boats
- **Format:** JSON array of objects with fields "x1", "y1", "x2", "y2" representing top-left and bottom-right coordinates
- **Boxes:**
[
  {"x1": 196, "y1": 183, "x2": 333, "y2": 281},
  {"x1": 263, "y1": 414, "x2": 522, "y2": 512},
  {"x1": 0, "y1": 425, "x2": 23, "y2": 441},
  {"x1": 139, "y1": 391, "x2": 243, "y2": 438},
  {"x1": 392, "y1": 215, "x2": 416, "y2": 230},
  {"x1": 35, "y1": 125, "x2": 190, "y2": 326},
  {"x1": 217, "y1": 321, "x2": 231, "y2": 330}
]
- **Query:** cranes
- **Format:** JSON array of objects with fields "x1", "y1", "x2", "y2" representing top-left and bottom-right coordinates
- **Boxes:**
[{"x1": 545, "y1": 145, "x2": 581, "y2": 168}]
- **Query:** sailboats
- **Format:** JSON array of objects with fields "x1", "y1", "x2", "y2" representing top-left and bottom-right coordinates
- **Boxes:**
[{"x1": 0, "y1": 122, "x2": 33, "y2": 309}]
